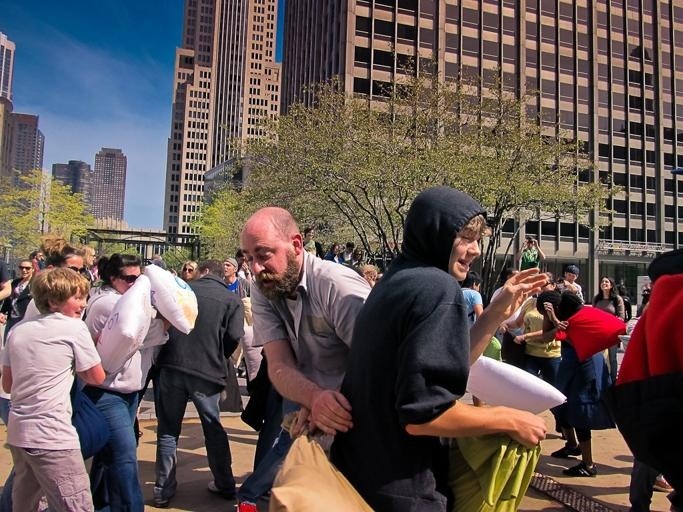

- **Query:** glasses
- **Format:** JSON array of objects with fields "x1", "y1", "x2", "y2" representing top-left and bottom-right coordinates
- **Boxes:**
[
  {"x1": 19, "y1": 265, "x2": 32, "y2": 270},
  {"x1": 544, "y1": 282, "x2": 550, "y2": 286},
  {"x1": 119, "y1": 274, "x2": 140, "y2": 283},
  {"x1": 526, "y1": 241, "x2": 533, "y2": 244},
  {"x1": 91, "y1": 254, "x2": 95, "y2": 257},
  {"x1": 347, "y1": 248, "x2": 352, "y2": 251},
  {"x1": 68, "y1": 265, "x2": 86, "y2": 273},
  {"x1": 183, "y1": 267, "x2": 194, "y2": 272}
]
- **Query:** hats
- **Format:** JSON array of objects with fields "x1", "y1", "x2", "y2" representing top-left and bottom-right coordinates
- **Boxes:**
[{"x1": 225, "y1": 257, "x2": 239, "y2": 268}]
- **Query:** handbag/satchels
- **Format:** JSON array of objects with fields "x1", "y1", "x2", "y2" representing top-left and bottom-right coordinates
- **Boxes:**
[{"x1": 467, "y1": 310, "x2": 476, "y2": 321}]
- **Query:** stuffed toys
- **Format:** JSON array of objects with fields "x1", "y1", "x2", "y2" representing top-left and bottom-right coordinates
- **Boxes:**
[{"x1": 360, "y1": 264, "x2": 378, "y2": 287}]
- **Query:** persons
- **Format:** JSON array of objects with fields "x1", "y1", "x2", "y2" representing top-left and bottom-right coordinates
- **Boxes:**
[
  {"x1": 1, "y1": 259, "x2": 34, "y2": 346},
  {"x1": 640, "y1": 282, "x2": 652, "y2": 306},
  {"x1": 81, "y1": 253, "x2": 178, "y2": 512},
  {"x1": 330, "y1": 186, "x2": 549, "y2": 512},
  {"x1": 92, "y1": 256, "x2": 110, "y2": 287},
  {"x1": 221, "y1": 257, "x2": 264, "y2": 382},
  {"x1": 181, "y1": 261, "x2": 198, "y2": 281},
  {"x1": 237, "y1": 257, "x2": 251, "y2": 283},
  {"x1": 343, "y1": 241, "x2": 355, "y2": 265},
  {"x1": 324, "y1": 242, "x2": 342, "y2": 264},
  {"x1": 0, "y1": 348, "x2": 17, "y2": 512},
  {"x1": 603, "y1": 248, "x2": 683, "y2": 512},
  {"x1": 151, "y1": 257, "x2": 244, "y2": 507},
  {"x1": 490, "y1": 268, "x2": 533, "y2": 371},
  {"x1": 152, "y1": 258, "x2": 166, "y2": 269},
  {"x1": 499, "y1": 272, "x2": 560, "y2": 388},
  {"x1": 535, "y1": 291, "x2": 609, "y2": 478},
  {"x1": 29, "y1": 238, "x2": 99, "y2": 280},
  {"x1": 556, "y1": 264, "x2": 585, "y2": 305},
  {"x1": 592, "y1": 277, "x2": 625, "y2": 383},
  {"x1": 0, "y1": 266, "x2": 107, "y2": 512},
  {"x1": 302, "y1": 225, "x2": 324, "y2": 259},
  {"x1": 240, "y1": 206, "x2": 374, "y2": 438},
  {"x1": 0, "y1": 262, "x2": 11, "y2": 300},
  {"x1": 459, "y1": 271, "x2": 484, "y2": 326},
  {"x1": 519, "y1": 236, "x2": 546, "y2": 271}
]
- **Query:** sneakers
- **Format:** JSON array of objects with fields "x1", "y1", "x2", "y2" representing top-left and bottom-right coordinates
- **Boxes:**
[
  {"x1": 207, "y1": 478, "x2": 235, "y2": 499},
  {"x1": 653, "y1": 475, "x2": 674, "y2": 491},
  {"x1": 236, "y1": 497, "x2": 259, "y2": 512},
  {"x1": 563, "y1": 462, "x2": 598, "y2": 479},
  {"x1": 153, "y1": 489, "x2": 177, "y2": 508},
  {"x1": 550, "y1": 443, "x2": 581, "y2": 458}
]
